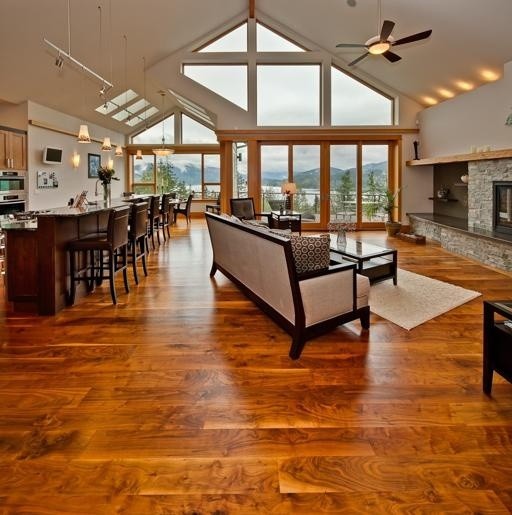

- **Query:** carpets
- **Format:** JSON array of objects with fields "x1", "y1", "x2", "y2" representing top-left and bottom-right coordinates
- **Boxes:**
[{"x1": 367, "y1": 267, "x2": 483, "y2": 332}]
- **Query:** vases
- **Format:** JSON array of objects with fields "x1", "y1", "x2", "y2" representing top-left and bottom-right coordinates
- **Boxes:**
[{"x1": 335, "y1": 229, "x2": 346, "y2": 244}]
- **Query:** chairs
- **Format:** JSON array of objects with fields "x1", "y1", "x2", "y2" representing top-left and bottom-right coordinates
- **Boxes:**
[
  {"x1": 230, "y1": 197, "x2": 272, "y2": 228},
  {"x1": 63, "y1": 188, "x2": 197, "y2": 306},
  {"x1": 330, "y1": 197, "x2": 389, "y2": 222}
]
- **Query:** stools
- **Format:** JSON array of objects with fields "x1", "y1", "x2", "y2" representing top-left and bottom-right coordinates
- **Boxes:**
[{"x1": 206, "y1": 204, "x2": 219, "y2": 214}]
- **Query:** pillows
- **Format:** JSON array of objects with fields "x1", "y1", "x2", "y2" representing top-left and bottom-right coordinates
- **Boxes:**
[
  {"x1": 267, "y1": 229, "x2": 331, "y2": 277},
  {"x1": 242, "y1": 217, "x2": 270, "y2": 230},
  {"x1": 231, "y1": 215, "x2": 243, "y2": 226},
  {"x1": 220, "y1": 211, "x2": 230, "y2": 221},
  {"x1": 269, "y1": 228, "x2": 292, "y2": 238}
]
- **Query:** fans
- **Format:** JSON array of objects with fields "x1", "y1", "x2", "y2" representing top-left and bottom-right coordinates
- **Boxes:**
[{"x1": 335, "y1": 0, "x2": 433, "y2": 67}]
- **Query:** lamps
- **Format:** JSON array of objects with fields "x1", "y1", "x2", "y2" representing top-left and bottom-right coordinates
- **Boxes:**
[
  {"x1": 236, "y1": 152, "x2": 242, "y2": 161},
  {"x1": 280, "y1": 181, "x2": 298, "y2": 211},
  {"x1": 40, "y1": 0, "x2": 175, "y2": 170},
  {"x1": 367, "y1": 42, "x2": 390, "y2": 56}
]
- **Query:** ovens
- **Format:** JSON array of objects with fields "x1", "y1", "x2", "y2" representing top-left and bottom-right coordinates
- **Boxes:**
[{"x1": 0, "y1": 170, "x2": 29, "y2": 215}]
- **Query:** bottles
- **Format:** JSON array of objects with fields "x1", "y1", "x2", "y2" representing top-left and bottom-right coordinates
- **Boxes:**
[{"x1": 279, "y1": 204, "x2": 284, "y2": 215}]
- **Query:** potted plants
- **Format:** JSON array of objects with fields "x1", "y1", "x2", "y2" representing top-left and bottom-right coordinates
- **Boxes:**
[
  {"x1": 364, "y1": 184, "x2": 410, "y2": 237},
  {"x1": 95, "y1": 165, "x2": 120, "y2": 202}
]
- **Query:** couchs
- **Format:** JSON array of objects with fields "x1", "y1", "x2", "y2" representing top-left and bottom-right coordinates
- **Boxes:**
[{"x1": 203, "y1": 209, "x2": 372, "y2": 362}]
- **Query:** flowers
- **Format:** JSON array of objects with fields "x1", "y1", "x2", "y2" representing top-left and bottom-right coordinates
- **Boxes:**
[{"x1": 325, "y1": 218, "x2": 359, "y2": 234}]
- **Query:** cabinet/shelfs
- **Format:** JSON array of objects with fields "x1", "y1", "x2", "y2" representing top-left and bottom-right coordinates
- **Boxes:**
[
  {"x1": 428, "y1": 182, "x2": 468, "y2": 204},
  {"x1": 482, "y1": 298, "x2": 512, "y2": 398},
  {"x1": 270, "y1": 210, "x2": 303, "y2": 236},
  {"x1": 0, "y1": 124, "x2": 28, "y2": 172}
]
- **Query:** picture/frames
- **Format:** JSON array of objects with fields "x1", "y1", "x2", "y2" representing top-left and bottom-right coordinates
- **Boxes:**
[{"x1": 87, "y1": 153, "x2": 102, "y2": 179}]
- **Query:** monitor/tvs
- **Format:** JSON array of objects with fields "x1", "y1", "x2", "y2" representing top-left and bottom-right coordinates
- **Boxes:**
[{"x1": 43, "y1": 146, "x2": 63, "y2": 165}]
